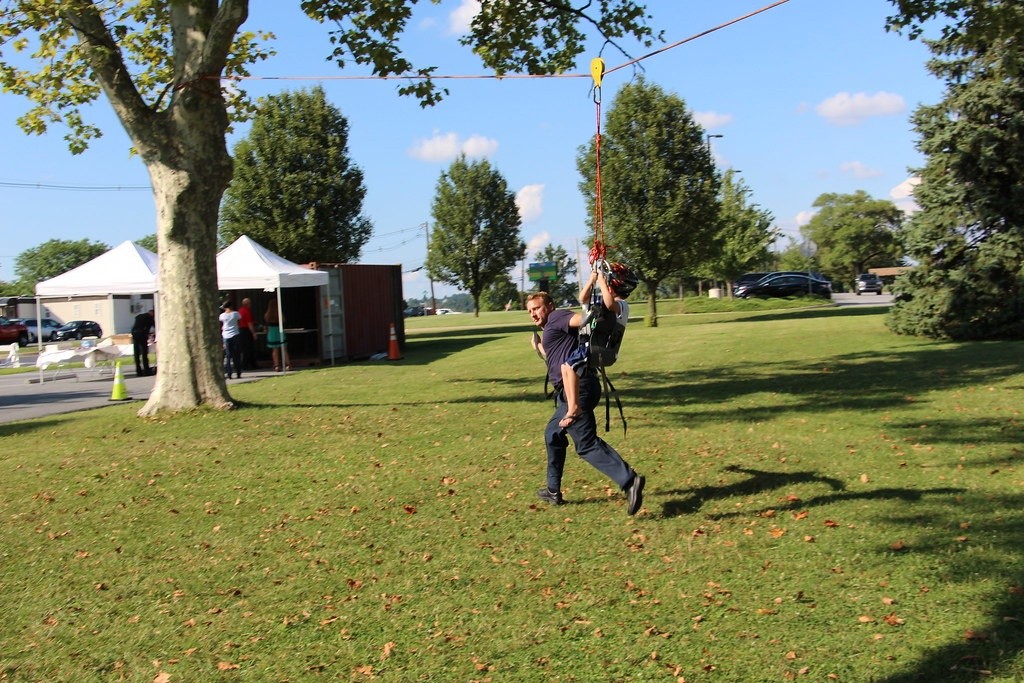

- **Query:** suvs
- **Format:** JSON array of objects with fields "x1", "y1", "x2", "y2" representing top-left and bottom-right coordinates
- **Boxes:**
[
  {"x1": 0, "y1": 315, "x2": 65, "y2": 348},
  {"x1": 855, "y1": 273, "x2": 884, "y2": 295}
]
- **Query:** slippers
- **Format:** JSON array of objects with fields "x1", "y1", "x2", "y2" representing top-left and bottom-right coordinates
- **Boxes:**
[{"x1": 560, "y1": 413, "x2": 585, "y2": 431}]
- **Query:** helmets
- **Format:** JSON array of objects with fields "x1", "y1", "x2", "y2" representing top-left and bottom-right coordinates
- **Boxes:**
[{"x1": 600, "y1": 263, "x2": 639, "y2": 298}]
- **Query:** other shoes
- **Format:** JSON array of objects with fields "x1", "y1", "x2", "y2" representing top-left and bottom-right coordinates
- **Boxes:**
[
  {"x1": 237, "y1": 373, "x2": 241, "y2": 378},
  {"x1": 275, "y1": 366, "x2": 281, "y2": 372},
  {"x1": 285, "y1": 365, "x2": 293, "y2": 371},
  {"x1": 226, "y1": 375, "x2": 231, "y2": 379}
]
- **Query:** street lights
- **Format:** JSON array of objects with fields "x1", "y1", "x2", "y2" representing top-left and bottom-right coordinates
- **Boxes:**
[{"x1": 707, "y1": 135, "x2": 723, "y2": 155}]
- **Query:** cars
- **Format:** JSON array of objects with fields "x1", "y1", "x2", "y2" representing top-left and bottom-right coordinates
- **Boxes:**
[
  {"x1": 57, "y1": 321, "x2": 103, "y2": 341},
  {"x1": 733, "y1": 271, "x2": 832, "y2": 300}
]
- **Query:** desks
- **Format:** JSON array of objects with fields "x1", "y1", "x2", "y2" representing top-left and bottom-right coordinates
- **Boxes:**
[
  {"x1": 251, "y1": 329, "x2": 318, "y2": 359},
  {"x1": 0, "y1": 343, "x2": 20, "y2": 368},
  {"x1": 37, "y1": 343, "x2": 156, "y2": 381}
]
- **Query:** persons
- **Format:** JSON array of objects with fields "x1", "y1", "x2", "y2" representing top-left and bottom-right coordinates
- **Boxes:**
[
  {"x1": 219, "y1": 300, "x2": 243, "y2": 379},
  {"x1": 238, "y1": 298, "x2": 257, "y2": 370},
  {"x1": 264, "y1": 299, "x2": 293, "y2": 371},
  {"x1": 132, "y1": 310, "x2": 155, "y2": 375},
  {"x1": 558, "y1": 262, "x2": 639, "y2": 427},
  {"x1": 525, "y1": 291, "x2": 646, "y2": 516}
]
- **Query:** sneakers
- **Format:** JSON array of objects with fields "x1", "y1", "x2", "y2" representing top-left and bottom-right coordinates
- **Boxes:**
[
  {"x1": 537, "y1": 488, "x2": 562, "y2": 504},
  {"x1": 626, "y1": 472, "x2": 646, "y2": 515}
]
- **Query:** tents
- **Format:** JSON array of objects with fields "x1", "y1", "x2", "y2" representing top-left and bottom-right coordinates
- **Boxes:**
[
  {"x1": 36, "y1": 240, "x2": 159, "y2": 385},
  {"x1": 216, "y1": 235, "x2": 334, "y2": 373}
]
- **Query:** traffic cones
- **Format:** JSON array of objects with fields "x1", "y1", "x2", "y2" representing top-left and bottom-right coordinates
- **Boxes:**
[
  {"x1": 107, "y1": 361, "x2": 134, "y2": 401},
  {"x1": 386, "y1": 323, "x2": 404, "y2": 360}
]
